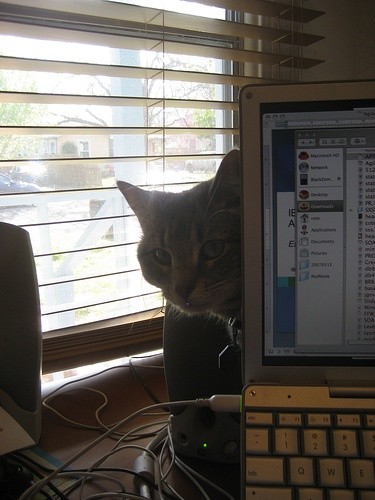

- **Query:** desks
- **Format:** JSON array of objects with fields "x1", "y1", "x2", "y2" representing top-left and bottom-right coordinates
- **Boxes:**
[{"x1": 1, "y1": 353, "x2": 238, "y2": 500}]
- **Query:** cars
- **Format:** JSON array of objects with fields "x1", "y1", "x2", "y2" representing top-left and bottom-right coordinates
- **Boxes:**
[{"x1": 0, "y1": 173, "x2": 43, "y2": 193}]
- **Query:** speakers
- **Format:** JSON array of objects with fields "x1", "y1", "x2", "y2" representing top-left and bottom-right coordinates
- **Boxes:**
[{"x1": 164, "y1": 300, "x2": 238, "y2": 462}]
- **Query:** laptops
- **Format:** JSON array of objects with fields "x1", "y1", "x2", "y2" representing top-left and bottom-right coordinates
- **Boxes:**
[
  {"x1": 0, "y1": 222, "x2": 44, "y2": 456},
  {"x1": 238, "y1": 79, "x2": 375, "y2": 499}
]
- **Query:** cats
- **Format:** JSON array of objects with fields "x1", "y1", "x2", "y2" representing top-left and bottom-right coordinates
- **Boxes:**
[{"x1": 116, "y1": 147, "x2": 245, "y2": 347}]
